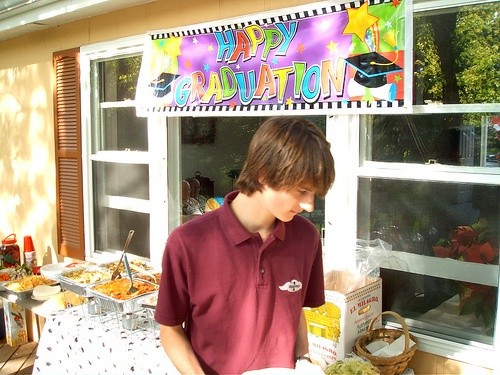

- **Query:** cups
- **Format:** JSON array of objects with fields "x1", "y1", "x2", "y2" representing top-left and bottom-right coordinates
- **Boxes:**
[{"x1": 24, "y1": 236, "x2": 35, "y2": 252}]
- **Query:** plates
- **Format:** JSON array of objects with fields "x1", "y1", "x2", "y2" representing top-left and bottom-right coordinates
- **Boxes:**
[{"x1": 30, "y1": 294, "x2": 48, "y2": 302}]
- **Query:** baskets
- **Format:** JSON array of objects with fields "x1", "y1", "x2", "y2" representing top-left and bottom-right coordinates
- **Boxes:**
[{"x1": 355, "y1": 311, "x2": 419, "y2": 375}]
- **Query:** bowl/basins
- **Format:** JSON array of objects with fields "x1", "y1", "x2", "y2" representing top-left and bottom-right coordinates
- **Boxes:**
[
  {"x1": 1, "y1": 275, "x2": 60, "y2": 299},
  {"x1": 0, "y1": 268, "x2": 34, "y2": 291},
  {"x1": 40, "y1": 262, "x2": 75, "y2": 280}
]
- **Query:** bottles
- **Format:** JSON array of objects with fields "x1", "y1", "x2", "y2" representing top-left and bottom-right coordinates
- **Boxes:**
[{"x1": 23, "y1": 249, "x2": 37, "y2": 268}]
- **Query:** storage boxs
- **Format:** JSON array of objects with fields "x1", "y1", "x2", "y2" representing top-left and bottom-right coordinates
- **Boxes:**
[
  {"x1": 3, "y1": 299, "x2": 29, "y2": 346},
  {"x1": 303, "y1": 271, "x2": 383, "y2": 364}
]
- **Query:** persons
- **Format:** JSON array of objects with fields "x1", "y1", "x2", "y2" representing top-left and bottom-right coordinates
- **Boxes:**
[
  {"x1": 154, "y1": 115, "x2": 336, "y2": 375},
  {"x1": 181, "y1": 180, "x2": 203, "y2": 215},
  {"x1": 185, "y1": 178, "x2": 208, "y2": 213}
]
